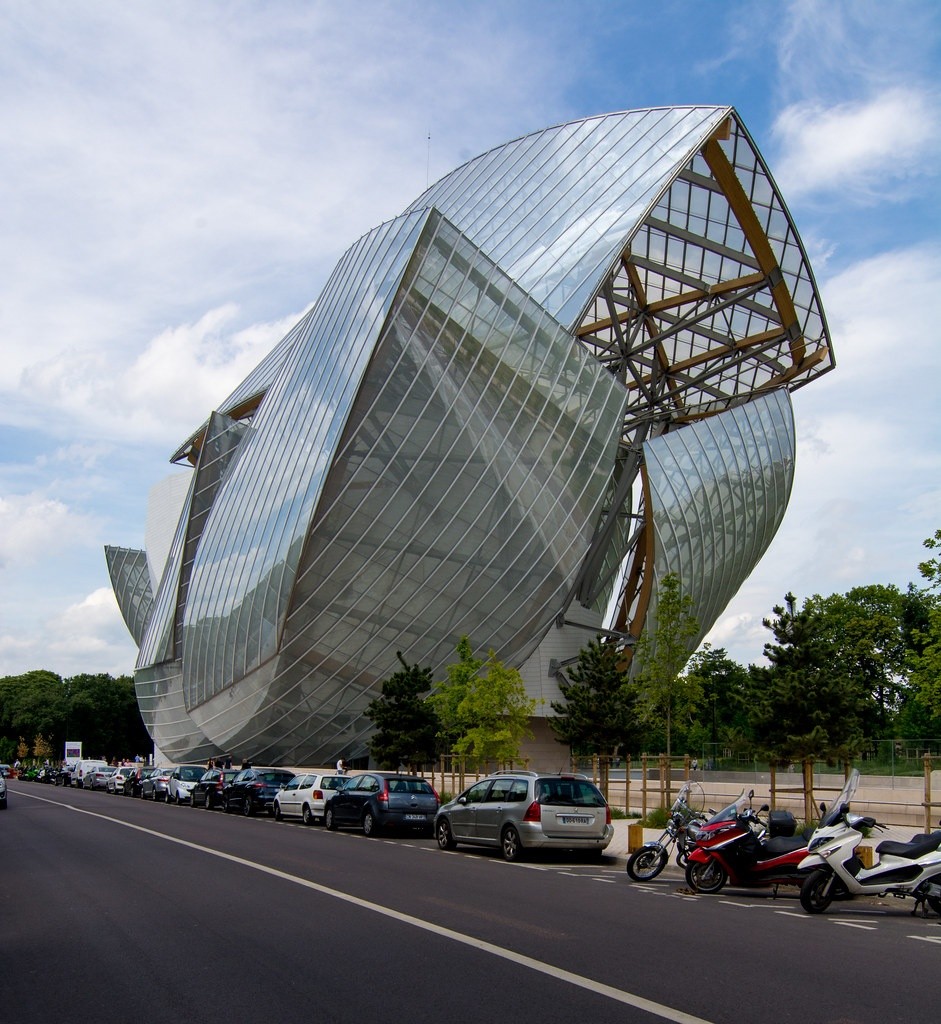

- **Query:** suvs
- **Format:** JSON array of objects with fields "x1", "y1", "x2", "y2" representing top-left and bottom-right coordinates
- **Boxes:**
[
  {"x1": 189, "y1": 768, "x2": 243, "y2": 810},
  {"x1": 222, "y1": 768, "x2": 295, "y2": 816},
  {"x1": 273, "y1": 772, "x2": 352, "y2": 827},
  {"x1": 433, "y1": 770, "x2": 614, "y2": 862}
]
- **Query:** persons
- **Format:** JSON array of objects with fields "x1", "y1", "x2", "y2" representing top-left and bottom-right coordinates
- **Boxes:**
[
  {"x1": 337, "y1": 756, "x2": 345, "y2": 784},
  {"x1": 43, "y1": 757, "x2": 49, "y2": 767},
  {"x1": 135, "y1": 753, "x2": 144, "y2": 763},
  {"x1": 112, "y1": 757, "x2": 132, "y2": 767},
  {"x1": 240, "y1": 759, "x2": 252, "y2": 770},
  {"x1": 692, "y1": 756, "x2": 698, "y2": 770},
  {"x1": 207, "y1": 758, "x2": 214, "y2": 770},
  {"x1": 609, "y1": 757, "x2": 614, "y2": 767},
  {"x1": 706, "y1": 756, "x2": 712, "y2": 770},
  {"x1": 14, "y1": 759, "x2": 21, "y2": 767},
  {"x1": 214, "y1": 757, "x2": 224, "y2": 770},
  {"x1": 615, "y1": 758, "x2": 620, "y2": 768},
  {"x1": 224, "y1": 753, "x2": 234, "y2": 770}
]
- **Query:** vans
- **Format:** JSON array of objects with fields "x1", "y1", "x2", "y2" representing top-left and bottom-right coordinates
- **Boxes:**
[
  {"x1": 71, "y1": 760, "x2": 109, "y2": 787},
  {"x1": 609, "y1": 756, "x2": 620, "y2": 767}
]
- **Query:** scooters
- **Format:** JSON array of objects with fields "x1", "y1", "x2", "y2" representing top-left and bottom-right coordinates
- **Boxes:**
[
  {"x1": 627, "y1": 781, "x2": 828, "y2": 901},
  {"x1": 797, "y1": 768, "x2": 941, "y2": 919}
]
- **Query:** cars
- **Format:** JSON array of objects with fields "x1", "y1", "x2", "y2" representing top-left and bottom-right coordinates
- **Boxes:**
[
  {"x1": 587, "y1": 756, "x2": 599, "y2": 765},
  {"x1": 323, "y1": 773, "x2": 440, "y2": 839},
  {"x1": 0, "y1": 761, "x2": 12, "y2": 809},
  {"x1": 18, "y1": 764, "x2": 77, "y2": 787},
  {"x1": 82, "y1": 766, "x2": 174, "y2": 801},
  {"x1": 165, "y1": 765, "x2": 209, "y2": 805}
]
